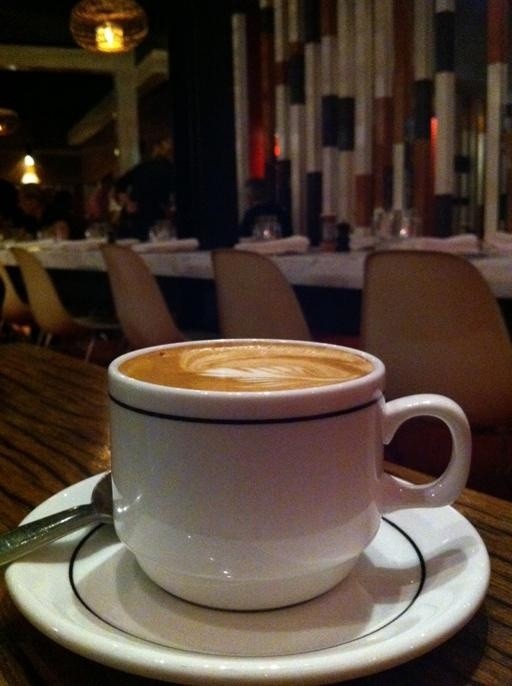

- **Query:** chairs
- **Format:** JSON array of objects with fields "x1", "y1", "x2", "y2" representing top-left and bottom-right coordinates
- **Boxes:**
[
  {"x1": 207, "y1": 246, "x2": 360, "y2": 355},
  {"x1": 362, "y1": 248, "x2": 511, "y2": 490},
  {"x1": 94, "y1": 245, "x2": 192, "y2": 350},
  {"x1": 0, "y1": 263, "x2": 33, "y2": 342},
  {"x1": 10, "y1": 246, "x2": 114, "y2": 365}
]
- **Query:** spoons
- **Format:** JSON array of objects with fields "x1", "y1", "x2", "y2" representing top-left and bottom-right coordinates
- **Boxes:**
[{"x1": 1, "y1": 468, "x2": 117, "y2": 569}]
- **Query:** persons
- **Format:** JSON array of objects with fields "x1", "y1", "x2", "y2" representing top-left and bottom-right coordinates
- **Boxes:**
[
  {"x1": 112, "y1": 128, "x2": 185, "y2": 242},
  {"x1": 83, "y1": 166, "x2": 113, "y2": 223},
  {"x1": 241, "y1": 176, "x2": 294, "y2": 239}
]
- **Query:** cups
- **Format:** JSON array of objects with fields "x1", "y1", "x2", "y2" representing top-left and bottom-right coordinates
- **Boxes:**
[{"x1": 106, "y1": 338, "x2": 474, "y2": 610}]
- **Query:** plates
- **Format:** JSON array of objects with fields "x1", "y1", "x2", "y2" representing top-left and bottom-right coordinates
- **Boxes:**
[{"x1": 6, "y1": 472, "x2": 492, "y2": 681}]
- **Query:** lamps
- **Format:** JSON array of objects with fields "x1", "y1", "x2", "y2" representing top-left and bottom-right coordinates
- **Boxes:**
[{"x1": 69, "y1": 0, "x2": 149, "y2": 58}]
